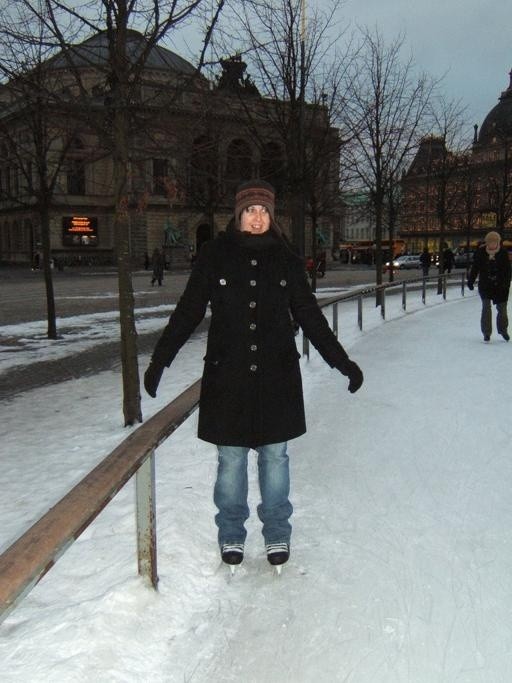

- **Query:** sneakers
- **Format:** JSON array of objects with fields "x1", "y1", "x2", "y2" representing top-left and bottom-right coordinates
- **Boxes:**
[
  {"x1": 502, "y1": 334, "x2": 510, "y2": 341},
  {"x1": 268, "y1": 541, "x2": 293, "y2": 566},
  {"x1": 483, "y1": 335, "x2": 491, "y2": 341},
  {"x1": 221, "y1": 543, "x2": 246, "y2": 566}
]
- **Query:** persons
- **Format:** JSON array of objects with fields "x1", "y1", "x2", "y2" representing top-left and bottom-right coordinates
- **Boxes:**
[
  {"x1": 144, "y1": 177, "x2": 365, "y2": 566},
  {"x1": 317, "y1": 255, "x2": 326, "y2": 277},
  {"x1": 437, "y1": 241, "x2": 456, "y2": 273},
  {"x1": 419, "y1": 246, "x2": 430, "y2": 280},
  {"x1": 466, "y1": 229, "x2": 511, "y2": 341},
  {"x1": 306, "y1": 255, "x2": 315, "y2": 278},
  {"x1": 149, "y1": 247, "x2": 164, "y2": 286}
]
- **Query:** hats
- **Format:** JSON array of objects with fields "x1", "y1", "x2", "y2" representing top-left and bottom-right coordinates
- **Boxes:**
[
  {"x1": 483, "y1": 231, "x2": 503, "y2": 244},
  {"x1": 232, "y1": 178, "x2": 277, "y2": 222}
]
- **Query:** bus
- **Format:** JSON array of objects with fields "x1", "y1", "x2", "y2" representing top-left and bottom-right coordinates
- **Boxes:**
[{"x1": 337, "y1": 238, "x2": 405, "y2": 260}]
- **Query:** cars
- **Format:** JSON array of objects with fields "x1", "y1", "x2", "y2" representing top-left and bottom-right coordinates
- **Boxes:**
[
  {"x1": 385, "y1": 255, "x2": 426, "y2": 270},
  {"x1": 435, "y1": 254, "x2": 472, "y2": 268}
]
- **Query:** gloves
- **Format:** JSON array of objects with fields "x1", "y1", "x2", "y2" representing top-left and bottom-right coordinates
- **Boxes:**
[
  {"x1": 336, "y1": 356, "x2": 364, "y2": 396},
  {"x1": 139, "y1": 359, "x2": 165, "y2": 400}
]
